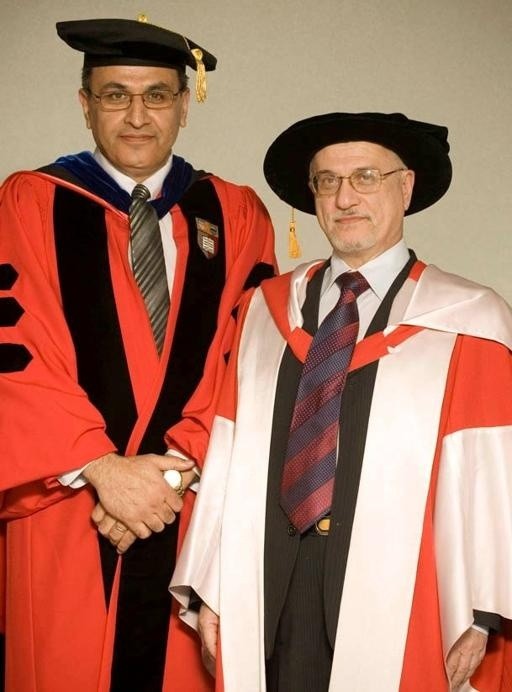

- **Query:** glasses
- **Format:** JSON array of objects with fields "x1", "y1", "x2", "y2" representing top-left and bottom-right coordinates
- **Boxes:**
[
  {"x1": 86, "y1": 88, "x2": 184, "y2": 111},
  {"x1": 308, "y1": 168, "x2": 410, "y2": 195}
]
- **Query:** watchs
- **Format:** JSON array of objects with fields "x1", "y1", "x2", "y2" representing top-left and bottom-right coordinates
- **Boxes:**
[{"x1": 162, "y1": 469, "x2": 185, "y2": 496}]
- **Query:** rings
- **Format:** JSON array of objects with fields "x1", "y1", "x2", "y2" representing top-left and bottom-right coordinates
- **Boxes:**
[{"x1": 115, "y1": 524, "x2": 126, "y2": 533}]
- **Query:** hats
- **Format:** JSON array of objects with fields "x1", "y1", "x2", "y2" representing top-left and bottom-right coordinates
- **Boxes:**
[
  {"x1": 55, "y1": 19, "x2": 219, "y2": 73},
  {"x1": 264, "y1": 112, "x2": 452, "y2": 218}
]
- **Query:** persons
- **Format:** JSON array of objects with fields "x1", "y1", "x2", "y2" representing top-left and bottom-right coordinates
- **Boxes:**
[
  {"x1": 0, "y1": 15, "x2": 281, "y2": 692},
  {"x1": 168, "y1": 112, "x2": 512, "y2": 692}
]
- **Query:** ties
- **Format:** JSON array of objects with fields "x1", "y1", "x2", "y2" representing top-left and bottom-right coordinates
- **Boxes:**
[
  {"x1": 279, "y1": 270, "x2": 372, "y2": 535},
  {"x1": 129, "y1": 183, "x2": 171, "y2": 356}
]
